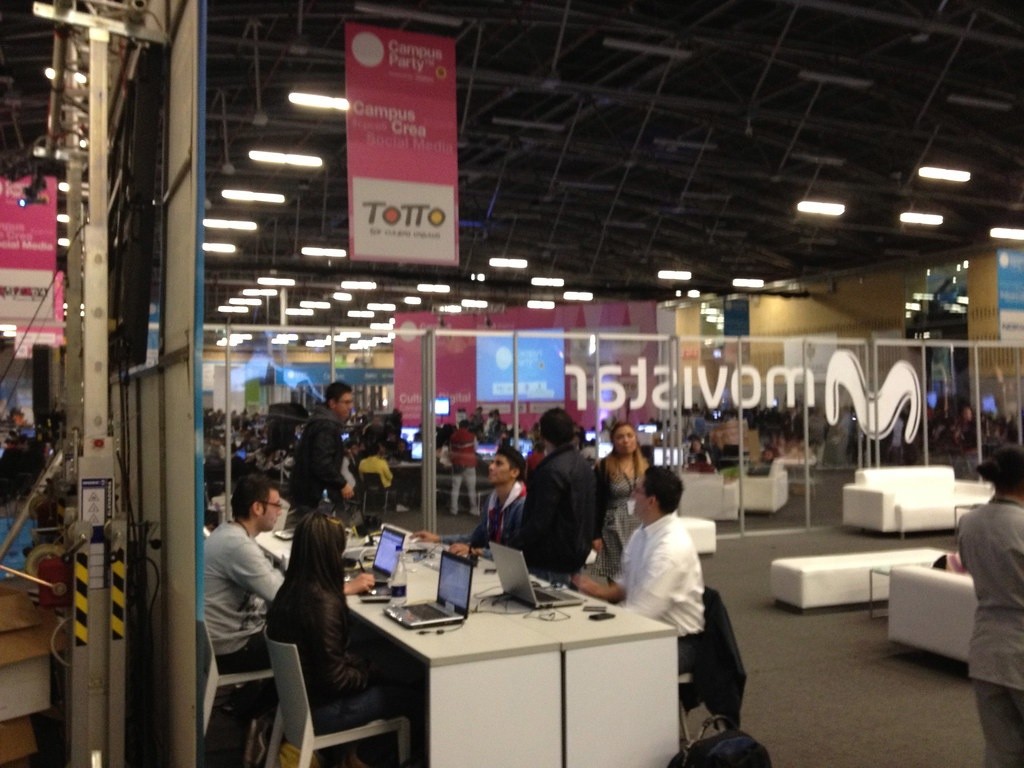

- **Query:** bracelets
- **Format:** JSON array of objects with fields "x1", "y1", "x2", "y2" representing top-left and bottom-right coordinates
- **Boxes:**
[{"x1": 469, "y1": 545, "x2": 472, "y2": 555}]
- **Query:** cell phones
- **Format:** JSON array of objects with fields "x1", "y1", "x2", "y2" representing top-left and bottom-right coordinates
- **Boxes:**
[
  {"x1": 361, "y1": 596, "x2": 391, "y2": 602},
  {"x1": 589, "y1": 613, "x2": 615, "y2": 622}
]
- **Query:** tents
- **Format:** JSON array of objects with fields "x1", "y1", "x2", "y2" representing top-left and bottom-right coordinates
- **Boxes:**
[{"x1": 230, "y1": 349, "x2": 326, "y2": 404}]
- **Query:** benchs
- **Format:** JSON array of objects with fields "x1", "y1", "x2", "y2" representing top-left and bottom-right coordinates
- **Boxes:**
[
  {"x1": 737, "y1": 459, "x2": 791, "y2": 513},
  {"x1": 841, "y1": 467, "x2": 995, "y2": 534},
  {"x1": 770, "y1": 547, "x2": 953, "y2": 615}
]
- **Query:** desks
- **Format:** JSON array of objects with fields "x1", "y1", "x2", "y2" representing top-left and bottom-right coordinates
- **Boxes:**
[
  {"x1": 256, "y1": 523, "x2": 683, "y2": 768},
  {"x1": 867, "y1": 563, "x2": 935, "y2": 627}
]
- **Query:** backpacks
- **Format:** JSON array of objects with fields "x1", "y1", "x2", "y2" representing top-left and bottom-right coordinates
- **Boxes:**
[{"x1": 683, "y1": 728, "x2": 774, "y2": 768}]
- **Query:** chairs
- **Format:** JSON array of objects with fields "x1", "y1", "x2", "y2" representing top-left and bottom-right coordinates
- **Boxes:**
[
  {"x1": 263, "y1": 625, "x2": 411, "y2": 768},
  {"x1": 203, "y1": 620, "x2": 274, "y2": 735},
  {"x1": 361, "y1": 473, "x2": 403, "y2": 517},
  {"x1": 885, "y1": 565, "x2": 980, "y2": 666},
  {"x1": 672, "y1": 473, "x2": 741, "y2": 522}
]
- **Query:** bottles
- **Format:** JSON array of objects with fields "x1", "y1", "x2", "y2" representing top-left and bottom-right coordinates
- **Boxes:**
[
  {"x1": 390, "y1": 545, "x2": 408, "y2": 606},
  {"x1": 318, "y1": 489, "x2": 330, "y2": 506}
]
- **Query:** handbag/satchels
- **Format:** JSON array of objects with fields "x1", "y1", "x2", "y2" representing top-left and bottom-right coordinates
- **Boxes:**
[
  {"x1": 242, "y1": 701, "x2": 279, "y2": 767},
  {"x1": 667, "y1": 713, "x2": 740, "y2": 768}
]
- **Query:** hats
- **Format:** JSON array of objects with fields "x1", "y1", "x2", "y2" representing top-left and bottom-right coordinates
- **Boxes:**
[{"x1": 497, "y1": 445, "x2": 527, "y2": 469}]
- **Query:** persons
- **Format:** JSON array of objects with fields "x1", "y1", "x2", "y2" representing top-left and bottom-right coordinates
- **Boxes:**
[
  {"x1": 506, "y1": 407, "x2": 603, "y2": 591},
  {"x1": 957, "y1": 444, "x2": 1024, "y2": 768},
  {"x1": 200, "y1": 409, "x2": 1024, "y2": 518},
  {"x1": 572, "y1": 466, "x2": 705, "y2": 676},
  {"x1": 286, "y1": 382, "x2": 355, "y2": 511},
  {"x1": 266, "y1": 512, "x2": 423, "y2": 768},
  {"x1": 203, "y1": 474, "x2": 376, "y2": 692},
  {"x1": 410, "y1": 446, "x2": 527, "y2": 560},
  {"x1": 591, "y1": 421, "x2": 651, "y2": 579}
]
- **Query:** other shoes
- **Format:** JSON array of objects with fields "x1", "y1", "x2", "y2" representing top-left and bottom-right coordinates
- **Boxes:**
[
  {"x1": 231, "y1": 686, "x2": 259, "y2": 703},
  {"x1": 396, "y1": 504, "x2": 409, "y2": 512},
  {"x1": 331, "y1": 744, "x2": 372, "y2": 768},
  {"x1": 469, "y1": 506, "x2": 480, "y2": 516},
  {"x1": 449, "y1": 506, "x2": 459, "y2": 516}
]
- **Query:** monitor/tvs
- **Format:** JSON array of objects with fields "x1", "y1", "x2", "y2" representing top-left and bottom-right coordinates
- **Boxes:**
[{"x1": 435, "y1": 398, "x2": 450, "y2": 416}]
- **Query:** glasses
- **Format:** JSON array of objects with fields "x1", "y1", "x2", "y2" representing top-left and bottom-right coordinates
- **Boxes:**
[
  {"x1": 490, "y1": 461, "x2": 504, "y2": 466},
  {"x1": 267, "y1": 502, "x2": 283, "y2": 509}
]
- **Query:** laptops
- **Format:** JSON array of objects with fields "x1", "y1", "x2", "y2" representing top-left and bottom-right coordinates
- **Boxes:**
[
  {"x1": 385, "y1": 550, "x2": 474, "y2": 628},
  {"x1": 489, "y1": 541, "x2": 583, "y2": 607},
  {"x1": 344, "y1": 527, "x2": 406, "y2": 585},
  {"x1": 400, "y1": 423, "x2": 684, "y2": 466}
]
